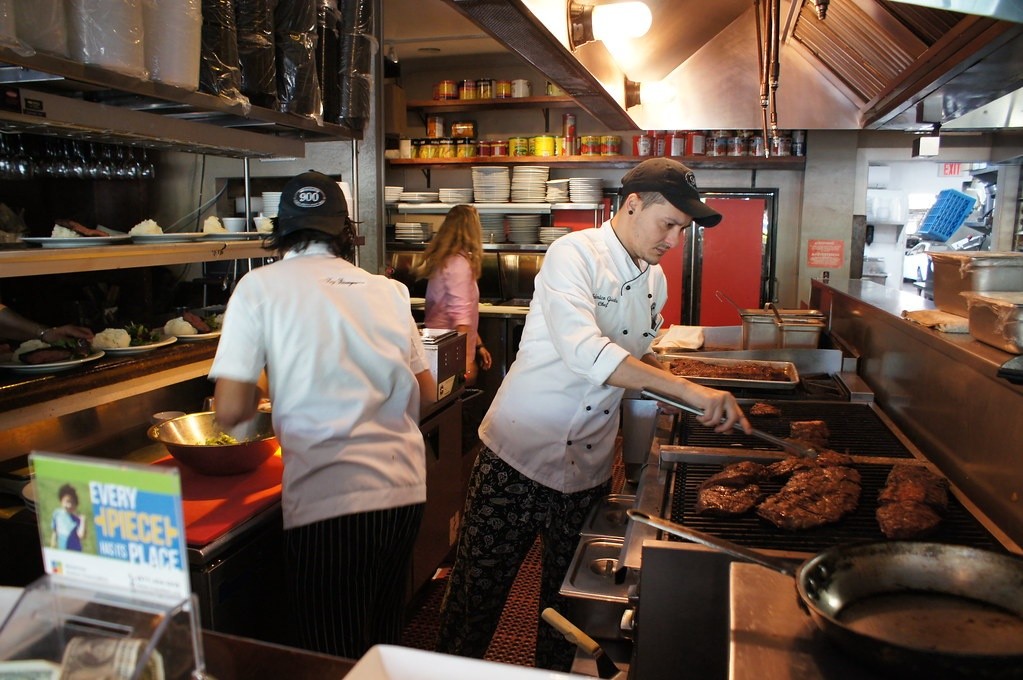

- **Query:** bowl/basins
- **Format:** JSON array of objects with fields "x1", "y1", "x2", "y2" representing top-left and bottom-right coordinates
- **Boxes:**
[
  {"x1": 152, "y1": 411, "x2": 187, "y2": 423},
  {"x1": 252, "y1": 217, "x2": 267, "y2": 231},
  {"x1": 147, "y1": 411, "x2": 280, "y2": 475},
  {"x1": 221, "y1": 217, "x2": 246, "y2": 233}
]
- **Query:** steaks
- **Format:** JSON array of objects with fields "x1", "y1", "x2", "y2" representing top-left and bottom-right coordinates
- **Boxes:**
[
  {"x1": 54, "y1": 219, "x2": 109, "y2": 236},
  {"x1": 695, "y1": 403, "x2": 953, "y2": 539},
  {"x1": 670, "y1": 358, "x2": 792, "y2": 382}
]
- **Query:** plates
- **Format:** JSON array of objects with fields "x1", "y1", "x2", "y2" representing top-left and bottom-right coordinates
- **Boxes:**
[
  {"x1": 104, "y1": 333, "x2": 178, "y2": 355},
  {"x1": 0, "y1": 348, "x2": 106, "y2": 374},
  {"x1": 19, "y1": 236, "x2": 132, "y2": 249},
  {"x1": 156, "y1": 327, "x2": 222, "y2": 342},
  {"x1": 206, "y1": 232, "x2": 264, "y2": 242},
  {"x1": 261, "y1": 191, "x2": 282, "y2": 219},
  {"x1": 131, "y1": 232, "x2": 208, "y2": 243},
  {"x1": 384, "y1": 164, "x2": 605, "y2": 246}
]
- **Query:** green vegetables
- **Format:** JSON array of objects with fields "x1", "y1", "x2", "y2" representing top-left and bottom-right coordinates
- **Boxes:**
[
  {"x1": 124, "y1": 321, "x2": 161, "y2": 346},
  {"x1": 53, "y1": 339, "x2": 91, "y2": 358},
  {"x1": 189, "y1": 308, "x2": 220, "y2": 328}
]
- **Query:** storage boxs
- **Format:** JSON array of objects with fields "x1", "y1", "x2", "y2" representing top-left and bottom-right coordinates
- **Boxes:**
[
  {"x1": 930, "y1": 253, "x2": 1022, "y2": 318},
  {"x1": 916, "y1": 189, "x2": 976, "y2": 242},
  {"x1": 743, "y1": 309, "x2": 826, "y2": 349},
  {"x1": 961, "y1": 293, "x2": 1023, "y2": 355}
]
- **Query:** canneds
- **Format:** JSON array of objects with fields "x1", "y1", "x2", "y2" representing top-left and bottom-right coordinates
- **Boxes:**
[
  {"x1": 632, "y1": 129, "x2": 806, "y2": 157},
  {"x1": 398, "y1": 77, "x2": 622, "y2": 157}
]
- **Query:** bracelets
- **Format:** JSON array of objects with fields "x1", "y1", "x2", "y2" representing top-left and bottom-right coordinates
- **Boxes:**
[
  {"x1": 41, "y1": 329, "x2": 48, "y2": 337},
  {"x1": 476, "y1": 344, "x2": 484, "y2": 349}
]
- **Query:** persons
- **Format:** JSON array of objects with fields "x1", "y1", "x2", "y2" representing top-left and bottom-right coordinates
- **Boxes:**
[
  {"x1": 0, "y1": 267, "x2": 145, "y2": 344},
  {"x1": 207, "y1": 168, "x2": 436, "y2": 660},
  {"x1": 410, "y1": 203, "x2": 492, "y2": 454},
  {"x1": 51, "y1": 484, "x2": 87, "y2": 551},
  {"x1": 432, "y1": 156, "x2": 752, "y2": 661}
]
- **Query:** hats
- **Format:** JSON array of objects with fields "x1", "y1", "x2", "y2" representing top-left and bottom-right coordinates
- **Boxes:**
[
  {"x1": 280, "y1": 168, "x2": 348, "y2": 233},
  {"x1": 618, "y1": 157, "x2": 722, "y2": 229}
]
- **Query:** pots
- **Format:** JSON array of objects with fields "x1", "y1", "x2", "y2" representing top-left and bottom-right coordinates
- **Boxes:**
[{"x1": 626, "y1": 507, "x2": 1023, "y2": 680}]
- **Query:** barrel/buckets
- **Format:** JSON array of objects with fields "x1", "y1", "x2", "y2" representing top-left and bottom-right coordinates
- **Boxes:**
[{"x1": 399, "y1": 77, "x2": 805, "y2": 158}]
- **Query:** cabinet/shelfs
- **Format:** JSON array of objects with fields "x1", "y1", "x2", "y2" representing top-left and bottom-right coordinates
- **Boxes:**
[
  {"x1": 1, "y1": 0, "x2": 376, "y2": 466},
  {"x1": 384, "y1": 93, "x2": 807, "y2": 266}
]
